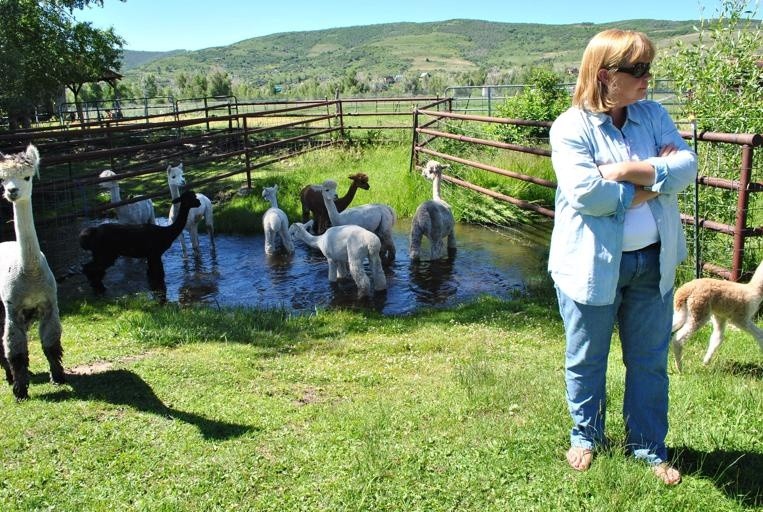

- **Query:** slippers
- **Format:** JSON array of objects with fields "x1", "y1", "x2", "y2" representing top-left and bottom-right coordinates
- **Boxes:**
[
  {"x1": 653, "y1": 463, "x2": 682, "y2": 487},
  {"x1": 565, "y1": 447, "x2": 594, "y2": 471}
]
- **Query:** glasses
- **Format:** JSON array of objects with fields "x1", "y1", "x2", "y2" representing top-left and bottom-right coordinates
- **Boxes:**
[{"x1": 607, "y1": 62, "x2": 651, "y2": 78}]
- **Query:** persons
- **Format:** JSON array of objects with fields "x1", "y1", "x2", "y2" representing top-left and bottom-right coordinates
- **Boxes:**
[
  {"x1": 105, "y1": 109, "x2": 113, "y2": 118},
  {"x1": 548, "y1": 29, "x2": 699, "y2": 487}
]
